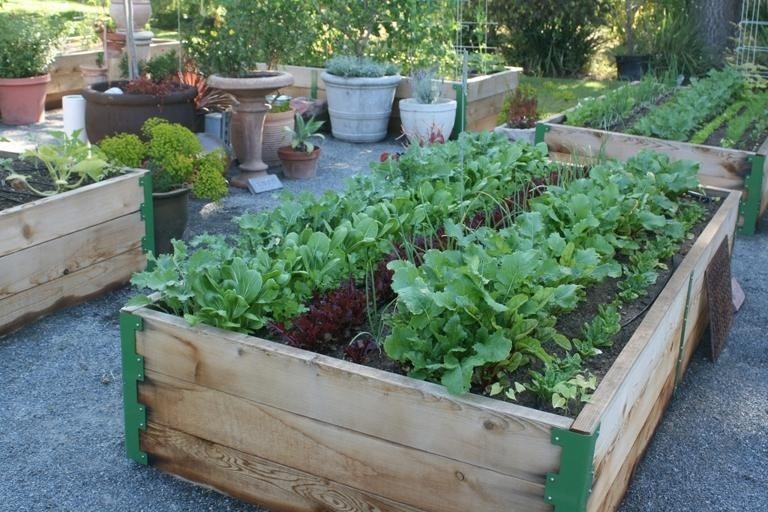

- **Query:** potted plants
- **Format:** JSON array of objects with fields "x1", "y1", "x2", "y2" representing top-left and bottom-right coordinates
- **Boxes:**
[{"x1": 653, "y1": 7, "x2": 709, "y2": 86}]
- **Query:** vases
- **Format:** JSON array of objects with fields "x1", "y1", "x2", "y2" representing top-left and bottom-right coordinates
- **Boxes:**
[{"x1": 615, "y1": 54, "x2": 649, "y2": 81}]
